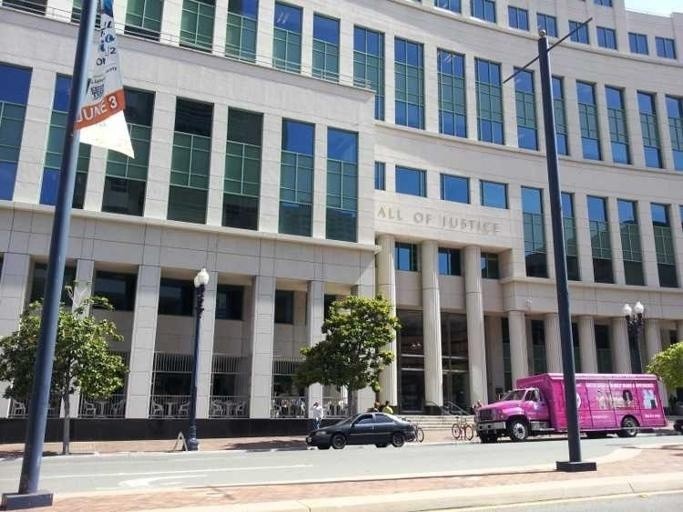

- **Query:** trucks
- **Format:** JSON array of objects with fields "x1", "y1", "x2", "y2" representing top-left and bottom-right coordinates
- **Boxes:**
[{"x1": 475, "y1": 370, "x2": 668, "y2": 442}]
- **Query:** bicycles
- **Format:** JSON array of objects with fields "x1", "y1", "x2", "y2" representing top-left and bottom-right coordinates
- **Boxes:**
[
  {"x1": 402, "y1": 418, "x2": 424, "y2": 443},
  {"x1": 451, "y1": 415, "x2": 474, "y2": 441}
]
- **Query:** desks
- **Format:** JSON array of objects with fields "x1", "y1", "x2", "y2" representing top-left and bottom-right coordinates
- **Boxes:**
[
  {"x1": 163, "y1": 402, "x2": 178, "y2": 417},
  {"x1": 94, "y1": 401, "x2": 109, "y2": 416}
]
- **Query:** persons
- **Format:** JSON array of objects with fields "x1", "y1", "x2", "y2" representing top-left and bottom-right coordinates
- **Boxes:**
[
  {"x1": 381, "y1": 400, "x2": 393, "y2": 415},
  {"x1": 367, "y1": 402, "x2": 380, "y2": 412},
  {"x1": 476, "y1": 400, "x2": 482, "y2": 411},
  {"x1": 645, "y1": 390, "x2": 656, "y2": 409},
  {"x1": 596, "y1": 390, "x2": 607, "y2": 410},
  {"x1": 309, "y1": 400, "x2": 323, "y2": 431},
  {"x1": 470, "y1": 404, "x2": 476, "y2": 415},
  {"x1": 668, "y1": 395, "x2": 678, "y2": 415},
  {"x1": 621, "y1": 389, "x2": 632, "y2": 408}
]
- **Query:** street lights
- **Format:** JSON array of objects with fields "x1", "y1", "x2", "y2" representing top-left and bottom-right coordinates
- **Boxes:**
[
  {"x1": 185, "y1": 267, "x2": 209, "y2": 451},
  {"x1": 623, "y1": 300, "x2": 644, "y2": 375}
]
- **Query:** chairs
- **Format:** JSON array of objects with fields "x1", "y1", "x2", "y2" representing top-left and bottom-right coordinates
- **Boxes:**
[
  {"x1": 272, "y1": 399, "x2": 305, "y2": 418},
  {"x1": 178, "y1": 401, "x2": 191, "y2": 416},
  {"x1": 47, "y1": 403, "x2": 55, "y2": 416},
  {"x1": 151, "y1": 399, "x2": 164, "y2": 417},
  {"x1": 211, "y1": 400, "x2": 246, "y2": 416},
  {"x1": 10, "y1": 399, "x2": 26, "y2": 417},
  {"x1": 110, "y1": 400, "x2": 125, "y2": 416},
  {"x1": 83, "y1": 399, "x2": 94, "y2": 416}
]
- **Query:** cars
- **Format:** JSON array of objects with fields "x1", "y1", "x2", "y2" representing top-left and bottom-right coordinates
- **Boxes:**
[{"x1": 304, "y1": 411, "x2": 415, "y2": 452}]
